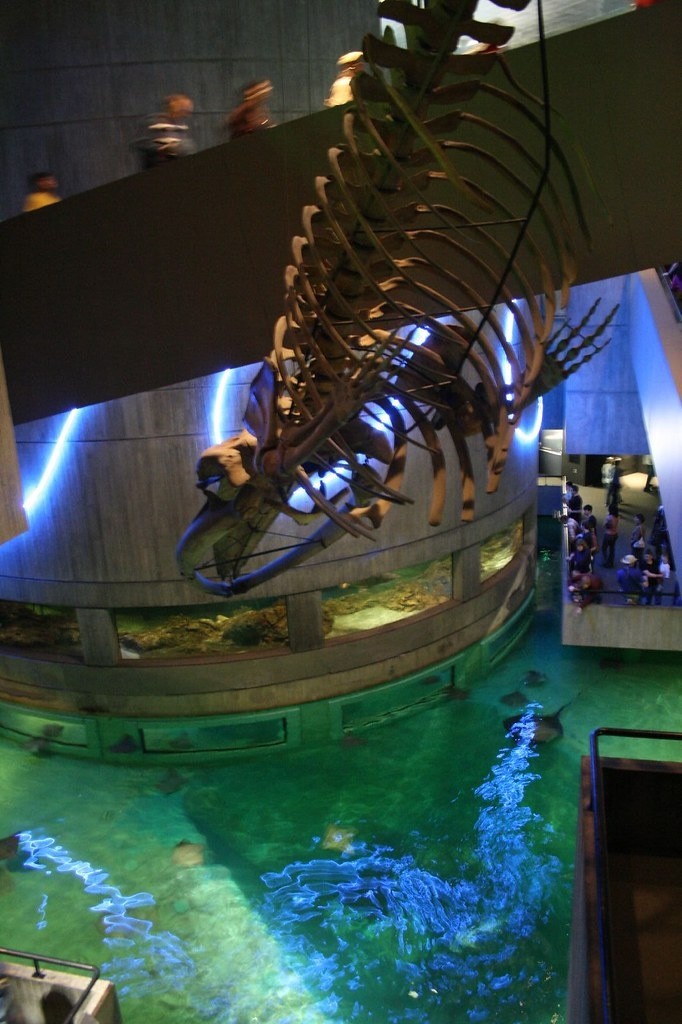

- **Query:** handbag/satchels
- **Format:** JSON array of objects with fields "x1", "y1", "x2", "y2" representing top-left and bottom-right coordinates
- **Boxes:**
[
  {"x1": 606, "y1": 518, "x2": 613, "y2": 528},
  {"x1": 634, "y1": 526, "x2": 645, "y2": 548},
  {"x1": 647, "y1": 535, "x2": 655, "y2": 545},
  {"x1": 642, "y1": 575, "x2": 649, "y2": 588},
  {"x1": 659, "y1": 561, "x2": 670, "y2": 579}
]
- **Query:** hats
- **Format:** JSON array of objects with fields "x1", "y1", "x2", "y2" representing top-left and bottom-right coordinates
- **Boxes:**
[
  {"x1": 579, "y1": 576, "x2": 591, "y2": 585},
  {"x1": 160, "y1": 95, "x2": 193, "y2": 114},
  {"x1": 576, "y1": 539, "x2": 584, "y2": 545},
  {"x1": 243, "y1": 80, "x2": 273, "y2": 101},
  {"x1": 337, "y1": 51, "x2": 363, "y2": 64},
  {"x1": 620, "y1": 555, "x2": 639, "y2": 564}
]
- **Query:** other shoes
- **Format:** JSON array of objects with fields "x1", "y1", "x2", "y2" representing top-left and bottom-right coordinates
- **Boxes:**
[{"x1": 656, "y1": 584, "x2": 664, "y2": 592}]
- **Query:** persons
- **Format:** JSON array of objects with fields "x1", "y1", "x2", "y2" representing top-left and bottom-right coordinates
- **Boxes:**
[
  {"x1": 22, "y1": 50, "x2": 362, "y2": 215},
  {"x1": 560, "y1": 454, "x2": 671, "y2": 618}
]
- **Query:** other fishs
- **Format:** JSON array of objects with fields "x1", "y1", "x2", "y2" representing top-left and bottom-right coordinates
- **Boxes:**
[
  {"x1": 503, "y1": 701, "x2": 567, "y2": 747},
  {"x1": 321, "y1": 825, "x2": 359, "y2": 856},
  {"x1": 0, "y1": 831, "x2": 19, "y2": 862},
  {"x1": 171, "y1": 839, "x2": 206, "y2": 866}
]
